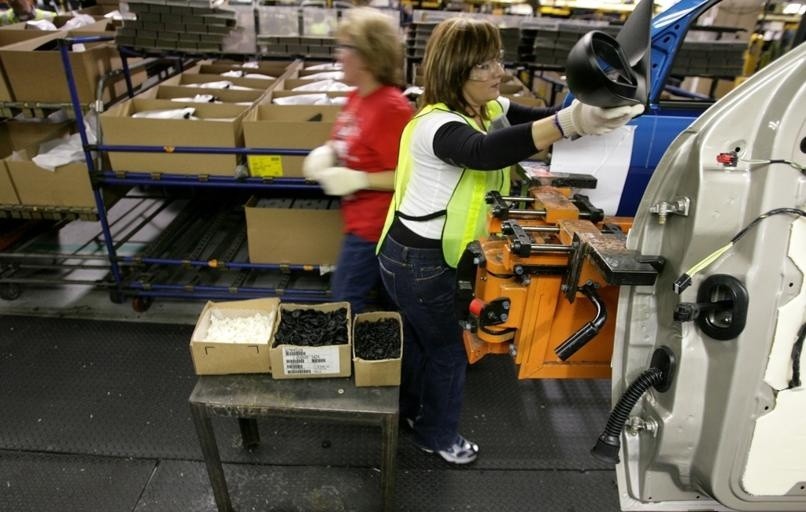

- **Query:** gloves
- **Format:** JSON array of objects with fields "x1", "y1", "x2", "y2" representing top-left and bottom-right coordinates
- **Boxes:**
[
  {"x1": 318, "y1": 166, "x2": 373, "y2": 197},
  {"x1": 554, "y1": 98, "x2": 645, "y2": 138},
  {"x1": 302, "y1": 144, "x2": 337, "y2": 182}
]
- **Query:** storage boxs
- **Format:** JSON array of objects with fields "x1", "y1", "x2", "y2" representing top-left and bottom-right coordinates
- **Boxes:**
[
  {"x1": 353, "y1": 311, "x2": 403, "y2": 388},
  {"x1": 1, "y1": 115, "x2": 101, "y2": 210},
  {"x1": 267, "y1": 300, "x2": 353, "y2": 378},
  {"x1": 499, "y1": 62, "x2": 571, "y2": 109},
  {"x1": 1, "y1": 1, "x2": 355, "y2": 181},
  {"x1": 188, "y1": 296, "x2": 280, "y2": 373},
  {"x1": 245, "y1": 183, "x2": 345, "y2": 265}
]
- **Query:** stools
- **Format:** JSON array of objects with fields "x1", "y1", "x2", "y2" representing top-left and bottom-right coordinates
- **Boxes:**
[{"x1": 188, "y1": 375, "x2": 401, "y2": 511}]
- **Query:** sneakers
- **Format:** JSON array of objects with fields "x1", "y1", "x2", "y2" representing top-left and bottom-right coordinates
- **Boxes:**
[{"x1": 418, "y1": 432, "x2": 479, "y2": 466}]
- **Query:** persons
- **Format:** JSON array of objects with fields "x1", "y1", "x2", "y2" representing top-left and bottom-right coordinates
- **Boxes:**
[
  {"x1": 376, "y1": 15, "x2": 646, "y2": 464},
  {"x1": 302, "y1": 6, "x2": 415, "y2": 309},
  {"x1": 0, "y1": 0, "x2": 58, "y2": 26}
]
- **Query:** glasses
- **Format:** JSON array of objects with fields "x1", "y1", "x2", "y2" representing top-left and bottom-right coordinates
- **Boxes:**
[
  {"x1": 469, "y1": 47, "x2": 505, "y2": 82},
  {"x1": 328, "y1": 43, "x2": 356, "y2": 56}
]
